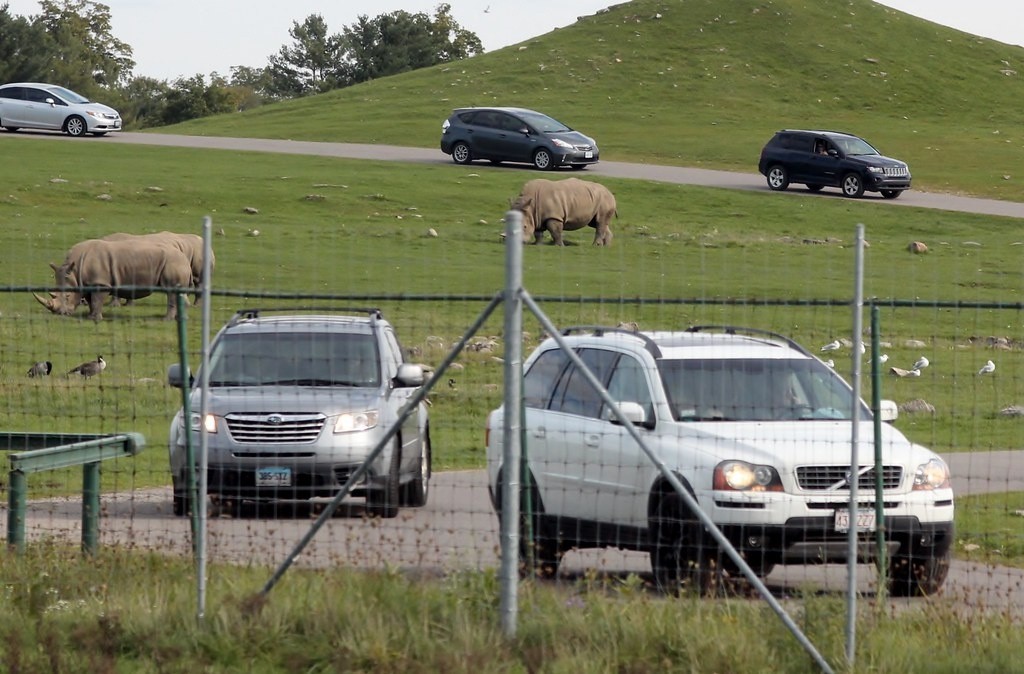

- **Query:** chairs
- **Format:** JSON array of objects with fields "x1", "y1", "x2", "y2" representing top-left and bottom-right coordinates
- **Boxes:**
[{"x1": 495, "y1": 116, "x2": 510, "y2": 129}]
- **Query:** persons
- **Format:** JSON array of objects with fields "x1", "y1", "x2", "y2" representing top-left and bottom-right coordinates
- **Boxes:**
[
  {"x1": 754, "y1": 375, "x2": 813, "y2": 420},
  {"x1": 819, "y1": 144, "x2": 828, "y2": 155}
]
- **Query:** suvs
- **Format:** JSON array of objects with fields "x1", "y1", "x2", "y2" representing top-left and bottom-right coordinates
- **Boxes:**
[
  {"x1": 165, "y1": 304, "x2": 435, "y2": 519},
  {"x1": 439, "y1": 107, "x2": 600, "y2": 171},
  {"x1": 757, "y1": 128, "x2": 913, "y2": 199},
  {"x1": 482, "y1": 322, "x2": 957, "y2": 600}
]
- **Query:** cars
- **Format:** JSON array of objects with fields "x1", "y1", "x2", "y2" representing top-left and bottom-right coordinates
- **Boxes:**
[{"x1": 0, "y1": 82, "x2": 121, "y2": 138}]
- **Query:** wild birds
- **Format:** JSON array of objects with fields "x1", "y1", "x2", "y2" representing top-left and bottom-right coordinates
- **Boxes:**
[
  {"x1": 66, "y1": 356, "x2": 107, "y2": 377},
  {"x1": 821, "y1": 324, "x2": 1024, "y2": 422},
  {"x1": 29, "y1": 361, "x2": 51, "y2": 377}
]
